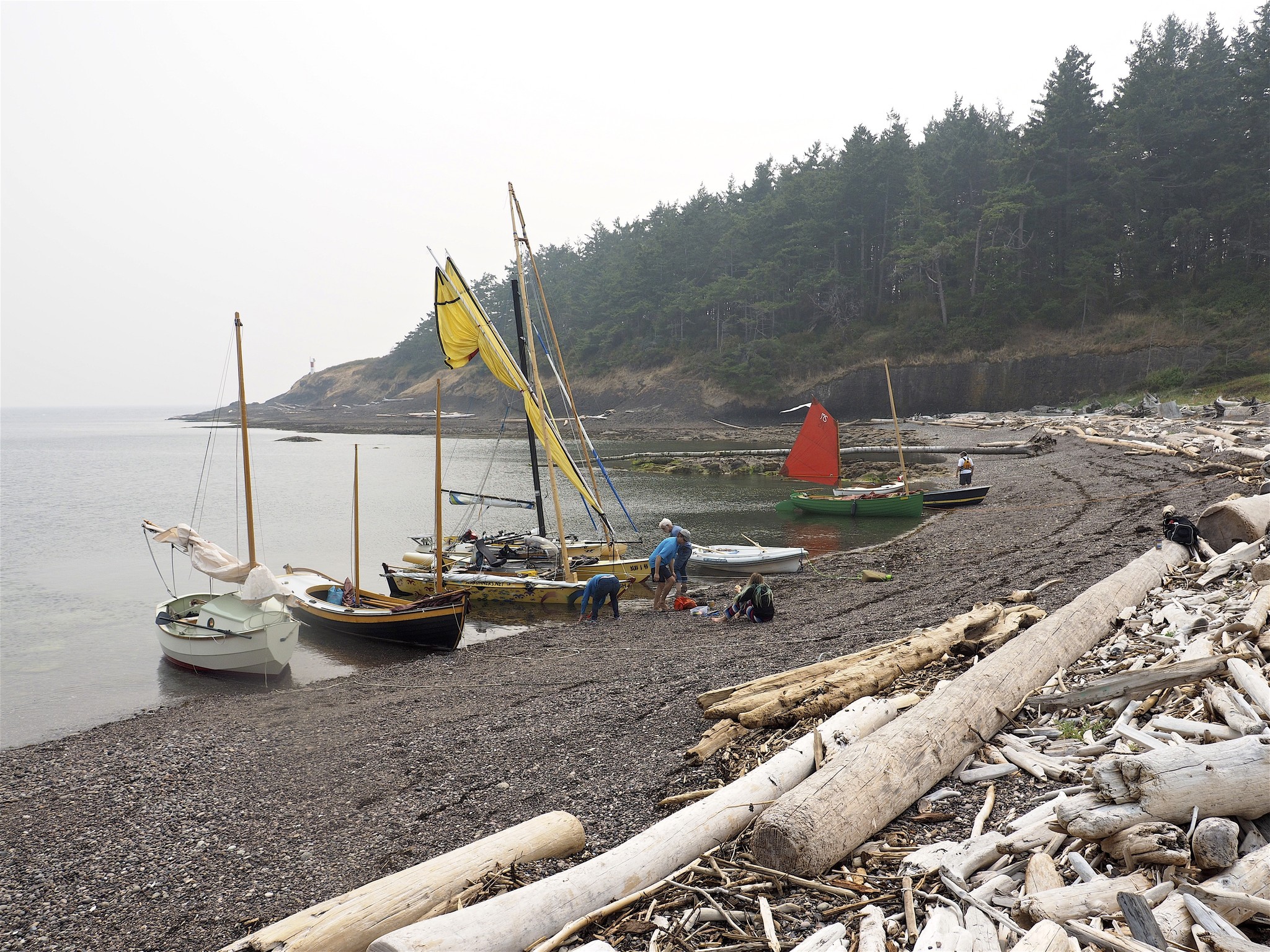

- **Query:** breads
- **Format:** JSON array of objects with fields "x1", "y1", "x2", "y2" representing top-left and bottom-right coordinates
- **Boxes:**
[{"x1": 735, "y1": 585, "x2": 742, "y2": 589}]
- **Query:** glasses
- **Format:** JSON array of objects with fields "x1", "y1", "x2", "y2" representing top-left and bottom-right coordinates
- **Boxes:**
[
  {"x1": 682, "y1": 536, "x2": 688, "y2": 542},
  {"x1": 661, "y1": 524, "x2": 668, "y2": 530}
]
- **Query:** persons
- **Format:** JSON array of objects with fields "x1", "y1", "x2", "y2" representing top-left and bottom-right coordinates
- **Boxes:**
[
  {"x1": 659, "y1": 518, "x2": 692, "y2": 595},
  {"x1": 649, "y1": 529, "x2": 691, "y2": 612},
  {"x1": 711, "y1": 573, "x2": 775, "y2": 623},
  {"x1": 956, "y1": 451, "x2": 974, "y2": 488},
  {"x1": 578, "y1": 574, "x2": 621, "y2": 623}
]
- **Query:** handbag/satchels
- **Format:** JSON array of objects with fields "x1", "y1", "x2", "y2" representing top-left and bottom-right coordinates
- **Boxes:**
[{"x1": 1162, "y1": 517, "x2": 1202, "y2": 546}]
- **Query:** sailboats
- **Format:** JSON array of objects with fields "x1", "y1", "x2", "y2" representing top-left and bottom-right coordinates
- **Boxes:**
[
  {"x1": 776, "y1": 394, "x2": 905, "y2": 496},
  {"x1": 790, "y1": 358, "x2": 924, "y2": 518},
  {"x1": 275, "y1": 377, "x2": 472, "y2": 654},
  {"x1": 156, "y1": 312, "x2": 301, "y2": 677},
  {"x1": 380, "y1": 188, "x2": 658, "y2": 607}
]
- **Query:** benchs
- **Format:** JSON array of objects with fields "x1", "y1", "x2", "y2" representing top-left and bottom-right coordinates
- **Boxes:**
[
  {"x1": 177, "y1": 616, "x2": 199, "y2": 624},
  {"x1": 706, "y1": 550, "x2": 738, "y2": 555}
]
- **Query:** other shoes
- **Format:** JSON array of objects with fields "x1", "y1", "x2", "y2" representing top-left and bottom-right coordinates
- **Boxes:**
[
  {"x1": 715, "y1": 590, "x2": 731, "y2": 597},
  {"x1": 695, "y1": 598, "x2": 716, "y2": 607},
  {"x1": 690, "y1": 593, "x2": 706, "y2": 598}
]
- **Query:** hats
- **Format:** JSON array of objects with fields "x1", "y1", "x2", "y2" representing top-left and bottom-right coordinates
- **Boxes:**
[
  {"x1": 959, "y1": 451, "x2": 967, "y2": 455},
  {"x1": 680, "y1": 529, "x2": 691, "y2": 542}
]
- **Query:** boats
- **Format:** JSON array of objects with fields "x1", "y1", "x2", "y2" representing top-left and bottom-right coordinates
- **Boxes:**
[
  {"x1": 685, "y1": 545, "x2": 809, "y2": 574},
  {"x1": 923, "y1": 485, "x2": 993, "y2": 508}
]
